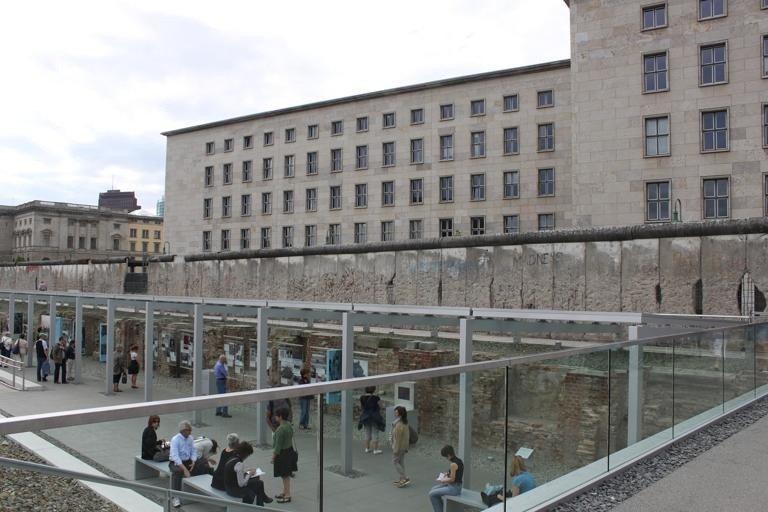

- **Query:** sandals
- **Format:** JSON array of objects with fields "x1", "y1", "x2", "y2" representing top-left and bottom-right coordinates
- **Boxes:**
[{"x1": 275, "y1": 494, "x2": 291, "y2": 503}]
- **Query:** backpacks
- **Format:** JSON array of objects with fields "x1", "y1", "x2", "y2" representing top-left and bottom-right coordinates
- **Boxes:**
[{"x1": 0, "y1": 338, "x2": 7, "y2": 351}]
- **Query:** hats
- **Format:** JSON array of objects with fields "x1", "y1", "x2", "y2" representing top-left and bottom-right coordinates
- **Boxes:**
[{"x1": 39, "y1": 333, "x2": 47, "y2": 337}]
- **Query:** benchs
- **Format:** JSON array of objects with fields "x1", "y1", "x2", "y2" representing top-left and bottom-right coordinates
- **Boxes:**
[
  {"x1": 439, "y1": 485, "x2": 493, "y2": 511},
  {"x1": 178, "y1": 471, "x2": 258, "y2": 512},
  {"x1": 132, "y1": 452, "x2": 188, "y2": 499}
]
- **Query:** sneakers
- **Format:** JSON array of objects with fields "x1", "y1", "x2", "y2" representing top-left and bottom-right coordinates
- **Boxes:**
[{"x1": 172, "y1": 497, "x2": 181, "y2": 507}]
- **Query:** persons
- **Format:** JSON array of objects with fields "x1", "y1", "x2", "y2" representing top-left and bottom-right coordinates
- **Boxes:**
[
  {"x1": 112, "y1": 343, "x2": 126, "y2": 393},
  {"x1": 427, "y1": 444, "x2": 465, "y2": 512},
  {"x1": 0, "y1": 331, "x2": 75, "y2": 385},
  {"x1": 481, "y1": 455, "x2": 537, "y2": 508},
  {"x1": 359, "y1": 386, "x2": 385, "y2": 454},
  {"x1": 390, "y1": 406, "x2": 411, "y2": 488},
  {"x1": 270, "y1": 407, "x2": 297, "y2": 503},
  {"x1": 213, "y1": 355, "x2": 232, "y2": 418},
  {"x1": 265, "y1": 397, "x2": 296, "y2": 452},
  {"x1": 128, "y1": 344, "x2": 140, "y2": 389},
  {"x1": 141, "y1": 414, "x2": 274, "y2": 508}
]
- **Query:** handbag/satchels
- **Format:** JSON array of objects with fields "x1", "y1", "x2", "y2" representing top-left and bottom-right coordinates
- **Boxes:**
[
  {"x1": 154, "y1": 448, "x2": 169, "y2": 461},
  {"x1": 122, "y1": 375, "x2": 128, "y2": 384},
  {"x1": 12, "y1": 343, "x2": 19, "y2": 354},
  {"x1": 408, "y1": 425, "x2": 418, "y2": 444},
  {"x1": 43, "y1": 362, "x2": 50, "y2": 374}
]
